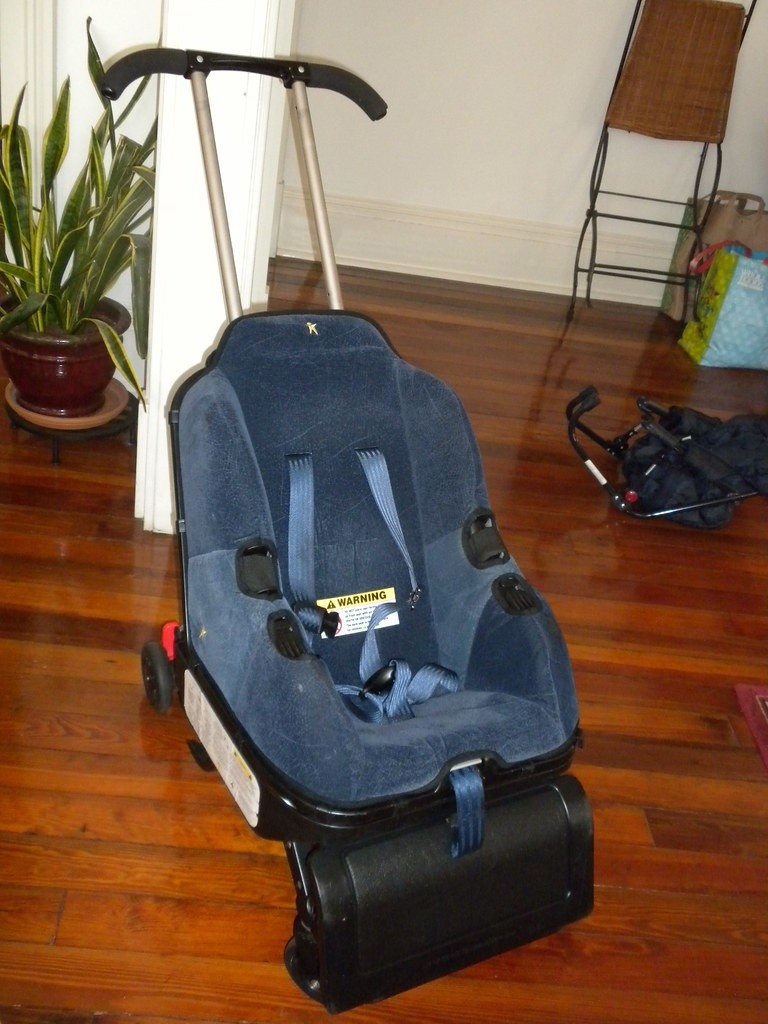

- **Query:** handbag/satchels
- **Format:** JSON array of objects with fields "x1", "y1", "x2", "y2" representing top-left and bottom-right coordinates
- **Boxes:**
[
  {"x1": 679, "y1": 241, "x2": 768, "y2": 373},
  {"x1": 659, "y1": 190, "x2": 768, "y2": 324}
]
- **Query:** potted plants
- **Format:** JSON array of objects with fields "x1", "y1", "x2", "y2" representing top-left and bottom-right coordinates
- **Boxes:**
[{"x1": 0, "y1": 17, "x2": 152, "y2": 417}]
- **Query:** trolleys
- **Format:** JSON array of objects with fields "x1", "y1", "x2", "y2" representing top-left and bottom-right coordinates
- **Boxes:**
[{"x1": 565, "y1": 383, "x2": 768, "y2": 529}]
